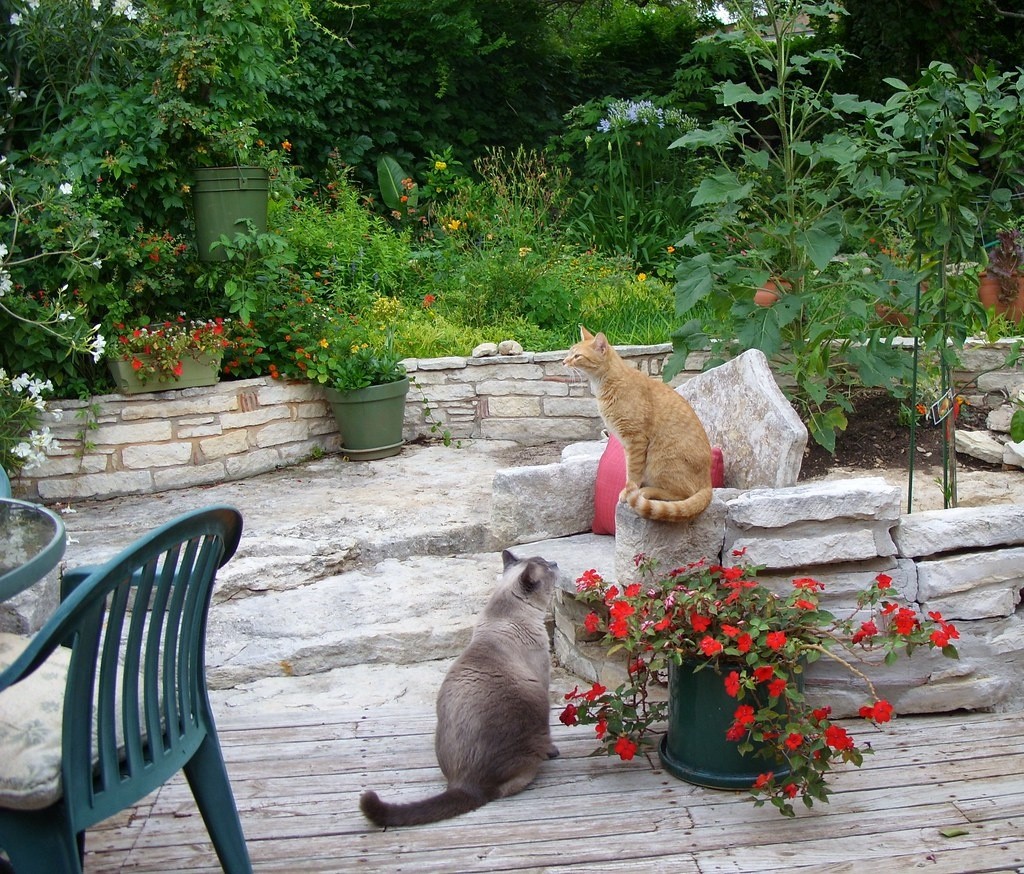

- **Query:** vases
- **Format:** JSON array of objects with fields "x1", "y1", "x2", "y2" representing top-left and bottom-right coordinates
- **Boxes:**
[
  {"x1": 319, "y1": 373, "x2": 411, "y2": 461},
  {"x1": 753, "y1": 279, "x2": 792, "y2": 307},
  {"x1": 976, "y1": 269, "x2": 1024, "y2": 324},
  {"x1": 876, "y1": 279, "x2": 928, "y2": 329},
  {"x1": 657, "y1": 648, "x2": 813, "y2": 794},
  {"x1": 104, "y1": 346, "x2": 224, "y2": 397}
]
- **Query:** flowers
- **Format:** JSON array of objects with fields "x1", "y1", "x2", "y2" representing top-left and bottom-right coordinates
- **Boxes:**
[
  {"x1": 102, "y1": 315, "x2": 230, "y2": 388},
  {"x1": 556, "y1": 540, "x2": 963, "y2": 818},
  {"x1": 295, "y1": 336, "x2": 478, "y2": 451}
]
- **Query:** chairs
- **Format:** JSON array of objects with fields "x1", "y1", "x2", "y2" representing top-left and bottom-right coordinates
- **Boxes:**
[
  {"x1": 489, "y1": 350, "x2": 809, "y2": 692},
  {"x1": 0, "y1": 505, "x2": 254, "y2": 874}
]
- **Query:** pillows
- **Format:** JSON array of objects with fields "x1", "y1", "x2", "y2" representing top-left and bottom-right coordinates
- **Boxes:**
[{"x1": 592, "y1": 432, "x2": 724, "y2": 537}]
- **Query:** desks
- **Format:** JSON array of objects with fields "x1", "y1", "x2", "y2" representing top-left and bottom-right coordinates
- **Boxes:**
[{"x1": 0, "y1": 496, "x2": 68, "y2": 606}]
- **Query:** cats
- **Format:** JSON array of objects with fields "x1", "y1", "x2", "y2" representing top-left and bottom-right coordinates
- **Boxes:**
[
  {"x1": 360, "y1": 548, "x2": 561, "y2": 827},
  {"x1": 563, "y1": 324, "x2": 711, "y2": 518}
]
